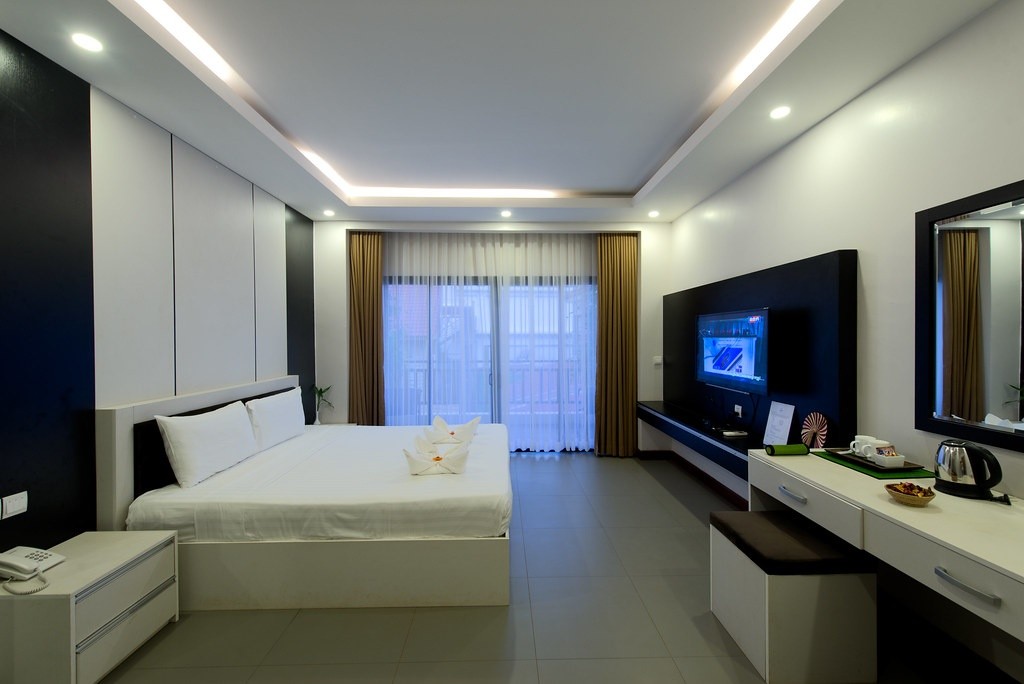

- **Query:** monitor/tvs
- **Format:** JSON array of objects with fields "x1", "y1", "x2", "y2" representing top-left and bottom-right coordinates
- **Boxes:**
[{"x1": 694, "y1": 308, "x2": 773, "y2": 399}]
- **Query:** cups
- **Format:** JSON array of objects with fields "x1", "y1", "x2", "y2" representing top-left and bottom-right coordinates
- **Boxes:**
[
  {"x1": 849, "y1": 435, "x2": 877, "y2": 458},
  {"x1": 861, "y1": 439, "x2": 890, "y2": 462}
]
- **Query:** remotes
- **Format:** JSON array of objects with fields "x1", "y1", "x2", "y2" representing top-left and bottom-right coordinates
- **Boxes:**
[{"x1": 723, "y1": 431, "x2": 748, "y2": 436}]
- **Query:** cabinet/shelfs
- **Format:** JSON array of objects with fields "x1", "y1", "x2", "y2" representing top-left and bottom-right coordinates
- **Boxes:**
[{"x1": 747, "y1": 448, "x2": 1024, "y2": 643}]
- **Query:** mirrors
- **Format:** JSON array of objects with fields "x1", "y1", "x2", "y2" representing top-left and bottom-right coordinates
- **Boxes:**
[{"x1": 911, "y1": 178, "x2": 1024, "y2": 455}]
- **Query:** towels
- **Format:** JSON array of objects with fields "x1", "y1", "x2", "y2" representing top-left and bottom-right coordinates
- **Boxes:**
[{"x1": 403, "y1": 415, "x2": 481, "y2": 476}]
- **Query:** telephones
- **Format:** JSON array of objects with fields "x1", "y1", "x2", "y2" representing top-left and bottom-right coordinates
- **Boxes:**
[{"x1": 0, "y1": 546, "x2": 67, "y2": 581}]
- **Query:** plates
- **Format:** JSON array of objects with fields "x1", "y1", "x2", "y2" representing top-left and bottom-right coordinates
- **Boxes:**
[{"x1": 825, "y1": 447, "x2": 925, "y2": 471}]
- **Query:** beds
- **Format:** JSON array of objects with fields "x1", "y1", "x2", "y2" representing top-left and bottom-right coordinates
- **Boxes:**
[{"x1": 124, "y1": 375, "x2": 513, "y2": 607}]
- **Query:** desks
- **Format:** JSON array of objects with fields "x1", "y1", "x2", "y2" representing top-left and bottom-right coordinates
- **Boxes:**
[{"x1": 635, "y1": 399, "x2": 769, "y2": 500}]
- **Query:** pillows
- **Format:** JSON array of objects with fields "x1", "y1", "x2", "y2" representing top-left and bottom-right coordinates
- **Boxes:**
[
  {"x1": 155, "y1": 401, "x2": 256, "y2": 487},
  {"x1": 245, "y1": 387, "x2": 304, "y2": 452}
]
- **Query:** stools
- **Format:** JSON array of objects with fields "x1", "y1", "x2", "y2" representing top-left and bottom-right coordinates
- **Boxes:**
[{"x1": 709, "y1": 509, "x2": 880, "y2": 684}]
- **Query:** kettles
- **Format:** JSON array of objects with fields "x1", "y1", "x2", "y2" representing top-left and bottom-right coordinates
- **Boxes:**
[{"x1": 934, "y1": 439, "x2": 1002, "y2": 495}]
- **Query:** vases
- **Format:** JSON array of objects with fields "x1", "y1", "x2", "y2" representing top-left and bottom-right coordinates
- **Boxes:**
[{"x1": 315, "y1": 411, "x2": 320, "y2": 426}]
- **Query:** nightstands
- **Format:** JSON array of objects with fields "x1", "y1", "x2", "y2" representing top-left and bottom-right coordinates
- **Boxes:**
[{"x1": 0, "y1": 530, "x2": 180, "y2": 684}]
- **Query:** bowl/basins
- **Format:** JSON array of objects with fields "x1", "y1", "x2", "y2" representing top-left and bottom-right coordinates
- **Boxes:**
[
  {"x1": 884, "y1": 482, "x2": 936, "y2": 507},
  {"x1": 874, "y1": 454, "x2": 904, "y2": 468}
]
